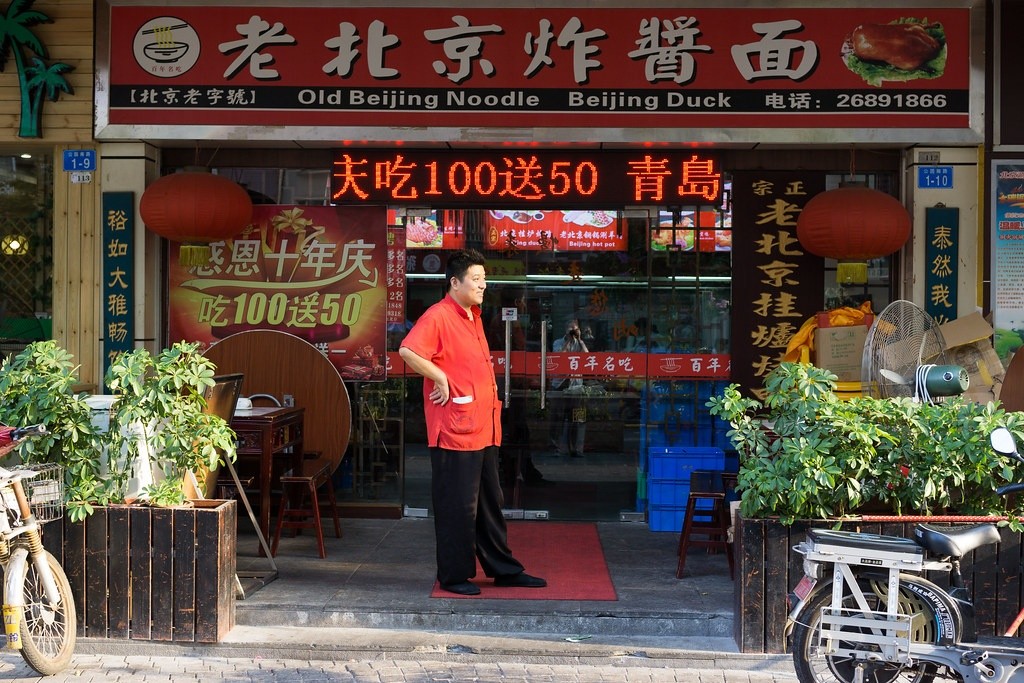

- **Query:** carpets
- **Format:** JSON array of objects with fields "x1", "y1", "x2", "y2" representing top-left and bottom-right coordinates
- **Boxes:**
[{"x1": 430, "y1": 518, "x2": 621, "y2": 601}]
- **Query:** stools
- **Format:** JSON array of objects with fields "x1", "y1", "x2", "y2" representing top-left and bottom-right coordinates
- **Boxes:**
[
  {"x1": 271, "y1": 459, "x2": 342, "y2": 560},
  {"x1": 676, "y1": 489, "x2": 735, "y2": 581}
]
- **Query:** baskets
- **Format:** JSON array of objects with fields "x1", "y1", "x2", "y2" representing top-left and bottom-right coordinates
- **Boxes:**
[{"x1": 0, "y1": 460, "x2": 65, "y2": 524}]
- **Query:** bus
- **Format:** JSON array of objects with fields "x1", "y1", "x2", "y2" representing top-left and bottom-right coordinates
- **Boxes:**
[{"x1": 399, "y1": 269, "x2": 735, "y2": 403}]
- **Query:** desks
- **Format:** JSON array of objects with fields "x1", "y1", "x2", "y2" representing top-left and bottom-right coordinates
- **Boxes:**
[
  {"x1": 827, "y1": 380, "x2": 885, "y2": 402},
  {"x1": 216, "y1": 406, "x2": 305, "y2": 558}
]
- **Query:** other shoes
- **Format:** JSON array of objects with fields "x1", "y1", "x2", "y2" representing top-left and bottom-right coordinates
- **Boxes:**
[
  {"x1": 494, "y1": 572, "x2": 548, "y2": 587},
  {"x1": 440, "y1": 580, "x2": 482, "y2": 595},
  {"x1": 570, "y1": 450, "x2": 584, "y2": 458},
  {"x1": 546, "y1": 443, "x2": 561, "y2": 452}
]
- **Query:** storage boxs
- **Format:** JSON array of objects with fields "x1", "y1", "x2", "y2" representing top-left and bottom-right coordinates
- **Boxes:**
[
  {"x1": 875, "y1": 312, "x2": 1006, "y2": 407},
  {"x1": 636, "y1": 347, "x2": 742, "y2": 530},
  {"x1": 814, "y1": 325, "x2": 872, "y2": 382}
]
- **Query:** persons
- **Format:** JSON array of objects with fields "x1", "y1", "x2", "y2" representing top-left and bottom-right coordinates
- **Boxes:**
[
  {"x1": 712, "y1": 338, "x2": 727, "y2": 353},
  {"x1": 397, "y1": 246, "x2": 549, "y2": 596},
  {"x1": 631, "y1": 318, "x2": 669, "y2": 355},
  {"x1": 549, "y1": 318, "x2": 590, "y2": 458},
  {"x1": 489, "y1": 281, "x2": 556, "y2": 493}
]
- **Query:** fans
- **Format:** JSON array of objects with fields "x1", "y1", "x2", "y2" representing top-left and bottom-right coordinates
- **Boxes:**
[{"x1": 858, "y1": 299, "x2": 970, "y2": 407}]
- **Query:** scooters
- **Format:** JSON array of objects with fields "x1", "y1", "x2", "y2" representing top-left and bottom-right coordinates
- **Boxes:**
[
  {"x1": 780, "y1": 427, "x2": 1024, "y2": 683},
  {"x1": 0, "y1": 421, "x2": 78, "y2": 678}
]
